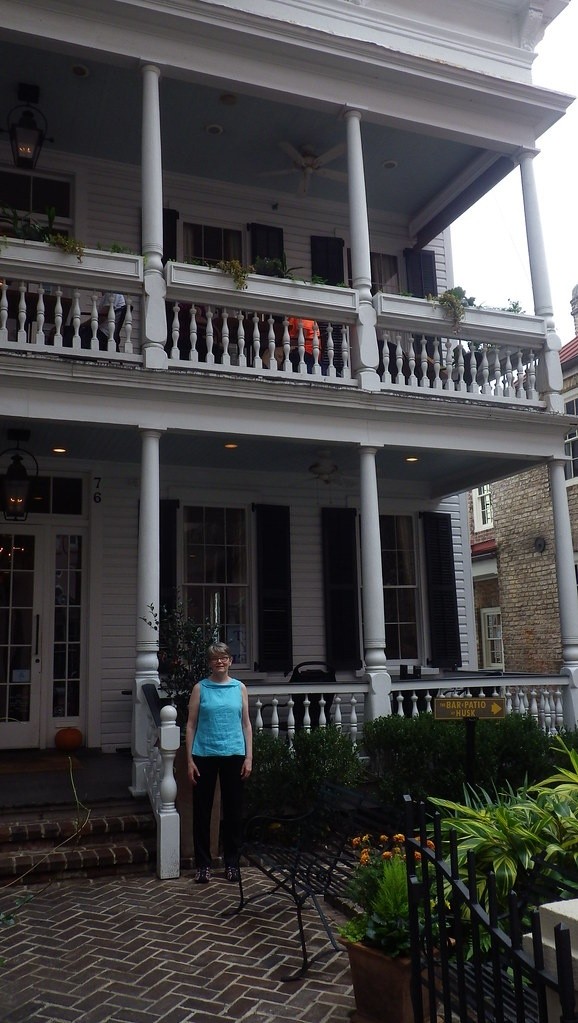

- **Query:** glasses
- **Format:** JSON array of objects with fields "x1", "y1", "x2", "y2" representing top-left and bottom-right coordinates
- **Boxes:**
[{"x1": 209, "y1": 657, "x2": 230, "y2": 663}]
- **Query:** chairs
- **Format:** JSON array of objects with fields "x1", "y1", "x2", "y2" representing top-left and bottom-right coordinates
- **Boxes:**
[
  {"x1": 259, "y1": 661, "x2": 337, "y2": 730},
  {"x1": 71, "y1": 305, "x2": 134, "y2": 352}
]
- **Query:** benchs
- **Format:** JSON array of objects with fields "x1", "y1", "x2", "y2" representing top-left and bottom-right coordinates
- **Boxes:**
[{"x1": 221, "y1": 777, "x2": 406, "y2": 985}]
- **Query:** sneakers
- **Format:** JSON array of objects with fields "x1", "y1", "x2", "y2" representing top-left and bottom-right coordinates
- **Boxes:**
[
  {"x1": 225, "y1": 865, "x2": 242, "y2": 881},
  {"x1": 194, "y1": 866, "x2": 209, "y2": 883}
]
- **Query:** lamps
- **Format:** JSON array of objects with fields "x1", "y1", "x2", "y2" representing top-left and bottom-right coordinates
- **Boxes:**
[
  {"x1": 0, "y1": 82, "x2": 54, "y2": 169},
  {"x1": 0, "y1": 428, "x2": 39, "y2": 521}
]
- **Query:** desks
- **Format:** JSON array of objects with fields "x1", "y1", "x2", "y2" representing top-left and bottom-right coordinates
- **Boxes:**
[
  {"x1": 0, "y1": 289, "x2": 72, "y2": 343},
  {"x1": 203, "y1": 316, "x2": 293, "y2": 369}
]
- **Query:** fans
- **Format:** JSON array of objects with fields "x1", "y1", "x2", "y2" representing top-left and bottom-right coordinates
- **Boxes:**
[
  {"x1": 256, "y1": 139, "x2": 348, "y2": 197},
  {"x1": 279, "y1": 448, "x2": 383, "y2": 485}
]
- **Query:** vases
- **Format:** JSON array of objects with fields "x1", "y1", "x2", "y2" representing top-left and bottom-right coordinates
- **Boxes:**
[{"x1": 334, "y1": 936, "x2": 456, "y2": 1023}]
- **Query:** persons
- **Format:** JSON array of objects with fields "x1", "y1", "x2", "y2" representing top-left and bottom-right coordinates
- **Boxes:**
[
  {"x1": 185, "y1": 642, "x2": 254, "y2": 883},
  {"x1": 281, "y1": 317, "x2": 321, "y2": 372},
  {"x1": 81, "y1": 292, "x2": 126, "y2": 348}
]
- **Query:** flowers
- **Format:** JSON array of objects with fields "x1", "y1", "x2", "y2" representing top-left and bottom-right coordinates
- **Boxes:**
[{"x1": 334, "y1": 831, "x2": 455, "y2": 958}]
